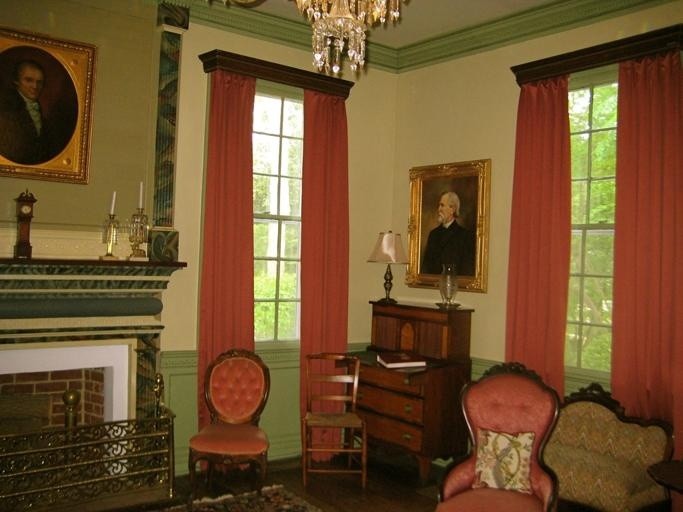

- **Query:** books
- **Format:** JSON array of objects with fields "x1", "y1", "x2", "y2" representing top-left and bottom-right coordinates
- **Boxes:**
[{"x1": 375, "y1": 350, "x2": 425, "y2": 370}]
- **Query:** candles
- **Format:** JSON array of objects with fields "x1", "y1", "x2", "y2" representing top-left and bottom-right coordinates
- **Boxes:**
[
  {"x1": 107, "y1": 188, "x2": 118, "y2": 217},
  {"x1": 135, "y1": 180, "x2": 147, "y2": 211}
]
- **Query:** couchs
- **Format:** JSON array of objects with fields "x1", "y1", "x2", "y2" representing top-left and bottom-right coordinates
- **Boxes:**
[
  {"x1": 434, "y1": 359, "x2": 562, "y2": 512},
  {"x1": 544, "y1": 382, "x2": 675, "y2": 511}
]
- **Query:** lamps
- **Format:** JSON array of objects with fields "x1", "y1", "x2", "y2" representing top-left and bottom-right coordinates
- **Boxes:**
[
  {"x1": 435, "y1": 263, "x2": 461, "y2": 310},
  {"x1": 295, "y1": 0, "x2": 401, "y2": 81},
  {"x1": 366, "y1": 231, "x2": 408, "y2": 306}
]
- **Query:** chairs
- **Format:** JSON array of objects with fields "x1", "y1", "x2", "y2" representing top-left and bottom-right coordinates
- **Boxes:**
[
  {"x1": 301, "y1": 350, "x2": 369, "y2": 493},
  {"x1": 185, "y1": 344, "x2": 275, "y2": 504}
]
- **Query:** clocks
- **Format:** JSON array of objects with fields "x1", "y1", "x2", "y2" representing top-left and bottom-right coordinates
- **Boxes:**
[{"x1": 12, "y1": 188, "x2": 37, "y2": 259}]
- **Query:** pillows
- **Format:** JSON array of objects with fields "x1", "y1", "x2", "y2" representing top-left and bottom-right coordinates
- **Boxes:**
[{"x1": 471, "y1": 425, "x2": 538, "y2": 496}]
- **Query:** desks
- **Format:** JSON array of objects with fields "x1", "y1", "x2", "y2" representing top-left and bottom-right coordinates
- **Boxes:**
[{"x1": 644, "y1": 458, "x2": 683, "y2": 493}]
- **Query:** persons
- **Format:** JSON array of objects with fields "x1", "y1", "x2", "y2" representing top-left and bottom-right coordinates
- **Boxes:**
[
  {"x1": 0, "y1": 59, "x2": 57, "y2": 164},
  {"x1": 419, "y1": 192, "x2": 475, "y2": 277}
]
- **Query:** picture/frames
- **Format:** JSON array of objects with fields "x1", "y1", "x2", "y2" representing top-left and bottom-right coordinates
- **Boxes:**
[
  {"x1": 403, "y1": 158, "x2": 496, "y2": 293},
  {"x1": 0, "y1": 29, "x2": 97, "y2": 185}
]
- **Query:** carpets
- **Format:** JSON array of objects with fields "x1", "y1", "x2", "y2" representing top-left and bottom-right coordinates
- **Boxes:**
[{"x1": 157, "y1": 481, "x2": 334, "y2": 512}]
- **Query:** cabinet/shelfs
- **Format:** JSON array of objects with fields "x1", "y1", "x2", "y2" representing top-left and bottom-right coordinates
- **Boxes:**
[{"x1": 345, "y1": 299, "x2": 474, "y2": 491}]
